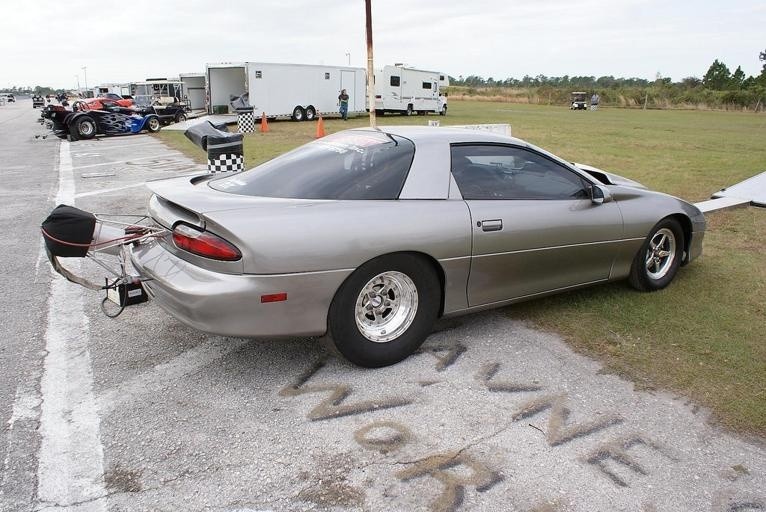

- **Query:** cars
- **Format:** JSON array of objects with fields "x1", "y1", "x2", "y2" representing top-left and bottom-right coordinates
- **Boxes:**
[
  {"x1": 0, "y1": 93, "x2": 15, "y2": 103},
  {"x1": 42, "y1": 92, "x2": 187, "y2": 140},
  {"x1": 128, "y1": 126, "x2": 707, "y2": 369},
  {"x1": 33, "y1": 97, "x2": 45, "y2": 108}
]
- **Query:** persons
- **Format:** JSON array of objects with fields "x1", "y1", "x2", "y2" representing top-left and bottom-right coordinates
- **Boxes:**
[
  {"x1": 7, "y1": 94, "x2": 15, "y2": 102},
  {"x1": 170, "y1": 93, "x2": 181, "y2": 102},
  {"x1": 574, "y1": 91, "x2": 600, "y2": 111},
  {"x1": 55, "y1": 91, "x2": 70, "y2": 108},
  {"x1": 32, "y1": 94, "x2": 44, "y2": 100},
  {"x1": 79, "y1": 93, "x2": 86, "y2": 99},
  {"x1": 338, "y1": 89, "x2": 349, "y2": 121},
  {"x1": 45, "y1": 95, "x2": 52, "y2": 102}
]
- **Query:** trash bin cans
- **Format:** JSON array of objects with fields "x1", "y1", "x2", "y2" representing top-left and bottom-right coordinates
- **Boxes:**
[
  {"x1": 591, "y1": 103, "x2": 597, "y2": 111},
  {"x1": 237, "y1": 110, "x2": 255, "y2": 133},
  {"x1": 207, "y1": 134, "x2": 245, "y2": 174}
]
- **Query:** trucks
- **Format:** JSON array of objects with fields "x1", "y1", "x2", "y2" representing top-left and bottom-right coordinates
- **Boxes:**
[{"x1": 366, "y1": 63, "x2": 448, "y2": 116}]
